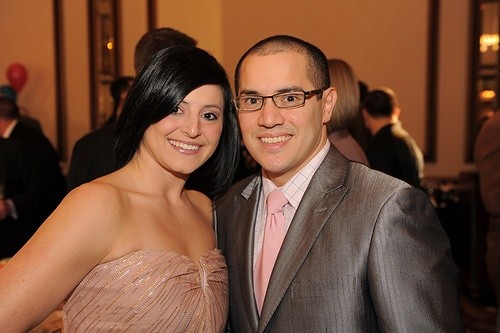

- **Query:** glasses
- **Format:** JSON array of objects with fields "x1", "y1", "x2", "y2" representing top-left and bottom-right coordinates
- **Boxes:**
[{"x1": 233, "y1": 87, "x2": 324, "y2": 111}]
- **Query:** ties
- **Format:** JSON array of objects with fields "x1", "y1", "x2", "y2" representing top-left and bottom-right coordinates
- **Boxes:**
[{"x1": 254, "y1": 190, "x2": 289, "y2": 317}]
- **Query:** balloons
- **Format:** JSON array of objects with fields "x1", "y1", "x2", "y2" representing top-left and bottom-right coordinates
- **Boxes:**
[{"x1": 6, "y1": 61, "x2": 29, "y2": 95}]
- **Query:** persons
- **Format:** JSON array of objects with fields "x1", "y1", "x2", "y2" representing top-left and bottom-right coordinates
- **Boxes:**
[
  {"x1": 474, "y1": 111, "x2": 500, "y2": 310},
  {"x1": 349, "y1": 81, "x2": 374, "y2": 156},
  {"x1": 0, "y1": 43, "x2": 237, "y2": 333},
  {"x1": 0, "y1": 84, "x2": 67, "y2": 260},
  {"x1": 65, "y1": 76, "x2": 135, "y2": 196},
  {"x1": 325, "y1": 58, "x2": 370, "y2": 169},
  {"x1": 361, "y1": 89, "x2": 424, "y2": 190},
  {"x1": 133, "y1": 27, "x2": 198, "y2": 77},
  {"x1": 216, "y1": 34, "x2": 468, "y2": 333}
]
